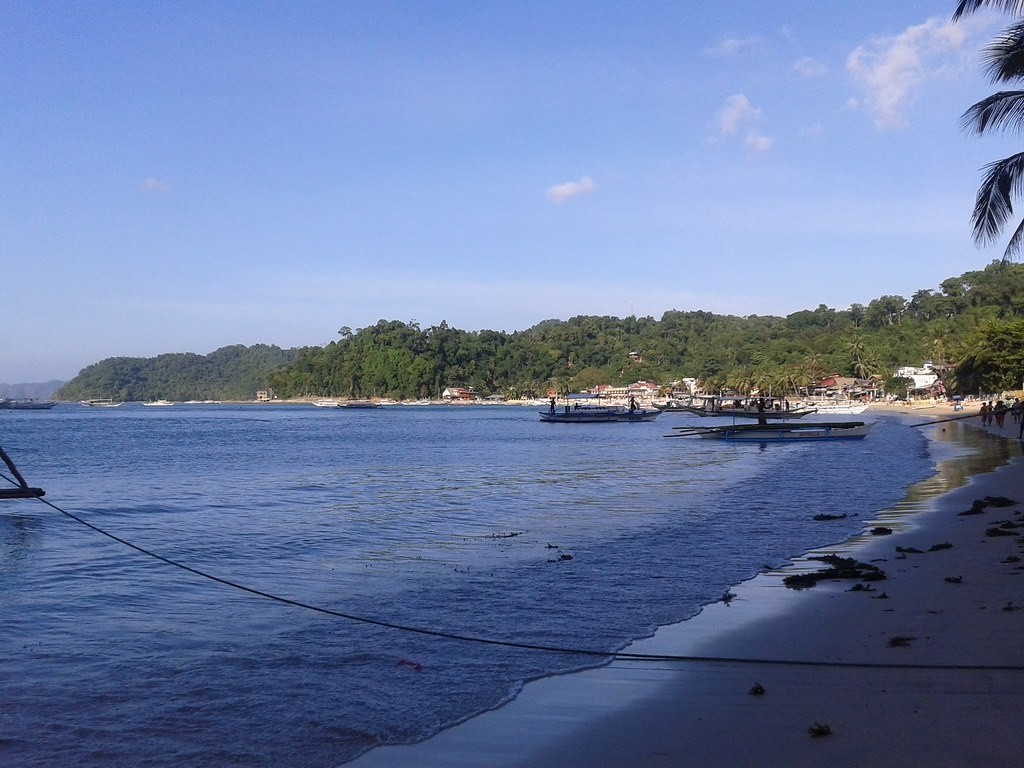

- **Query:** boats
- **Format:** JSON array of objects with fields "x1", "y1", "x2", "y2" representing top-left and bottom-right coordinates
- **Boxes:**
[
  {"x1": 539, "y1": 391, "x2": 663, "y2": 422},
  {"x1": 649, "y1": 392, "x2": 883, "y2": 441}
]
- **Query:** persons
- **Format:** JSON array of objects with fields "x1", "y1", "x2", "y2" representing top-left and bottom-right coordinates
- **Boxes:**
[
  {"x1": 550, "y1": 398, "x2": 556, "y2": 414},
  {"x1": 785, "y1": 399, "x2": 789, "y2": 411},
  {"x1": 1011, "y1": 397, "x2": 1024, "y2": 439},
  {"x1": 734, "y1": 400, "x2": 741, "y2": 408},
  {"x1": 629, "y1": 396, "x2": 638, "y2": 411},
  {"x1": 978, "y1": 401, "x2": 1008, "y2": 429}
]
